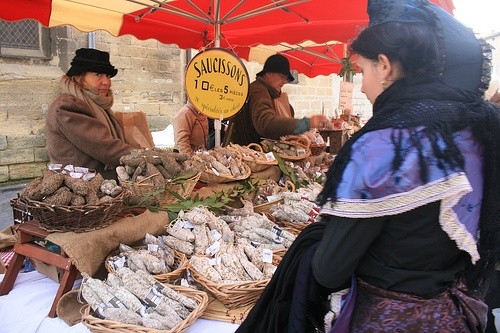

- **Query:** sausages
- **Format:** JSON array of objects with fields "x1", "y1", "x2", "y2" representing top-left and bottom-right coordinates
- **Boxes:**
[{"x1": 82, "y1": 182, "x2": 323, "y2": 330}]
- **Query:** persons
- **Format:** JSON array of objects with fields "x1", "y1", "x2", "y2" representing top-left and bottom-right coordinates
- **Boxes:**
[
  {"x1": 311, "y1": 0, "x2": 500, "y2": 333},
  {"x1": 45, "y1": 47, "x2": 146, "y2": 174},
  {"x1": 171, "y1": 95, "x2": 225, "y2": 155},
  {"x1": 222, "y1": 54, "x2": 329, "y2": 147}
]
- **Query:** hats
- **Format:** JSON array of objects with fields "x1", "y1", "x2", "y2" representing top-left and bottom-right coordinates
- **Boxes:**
[
  {"x1": 256, "y1": 54, "x2": 295, "y2": 83},
  {"x1": 66, "y1": 48, "x2": 118, "y2": 78}
]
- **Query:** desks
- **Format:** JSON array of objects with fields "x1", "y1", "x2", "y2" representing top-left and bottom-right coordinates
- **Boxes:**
[{"x1": 0, "y1": 225, "x2": 80, "y2": 318}]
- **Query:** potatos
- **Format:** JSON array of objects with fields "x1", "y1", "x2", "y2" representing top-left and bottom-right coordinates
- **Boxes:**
[{"x1": 22, "y1": 132, "x2": 337, "y2": 205}]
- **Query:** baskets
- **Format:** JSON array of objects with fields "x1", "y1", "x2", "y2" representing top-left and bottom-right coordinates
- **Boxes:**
[{"x1": 10, "y1": 136, "x2": 327, "y2": 333}]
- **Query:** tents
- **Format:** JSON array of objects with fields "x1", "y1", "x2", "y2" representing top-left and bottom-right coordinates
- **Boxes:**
[{"x1": 0, "y1": 0, "x2": 462, "y2": 148}]
